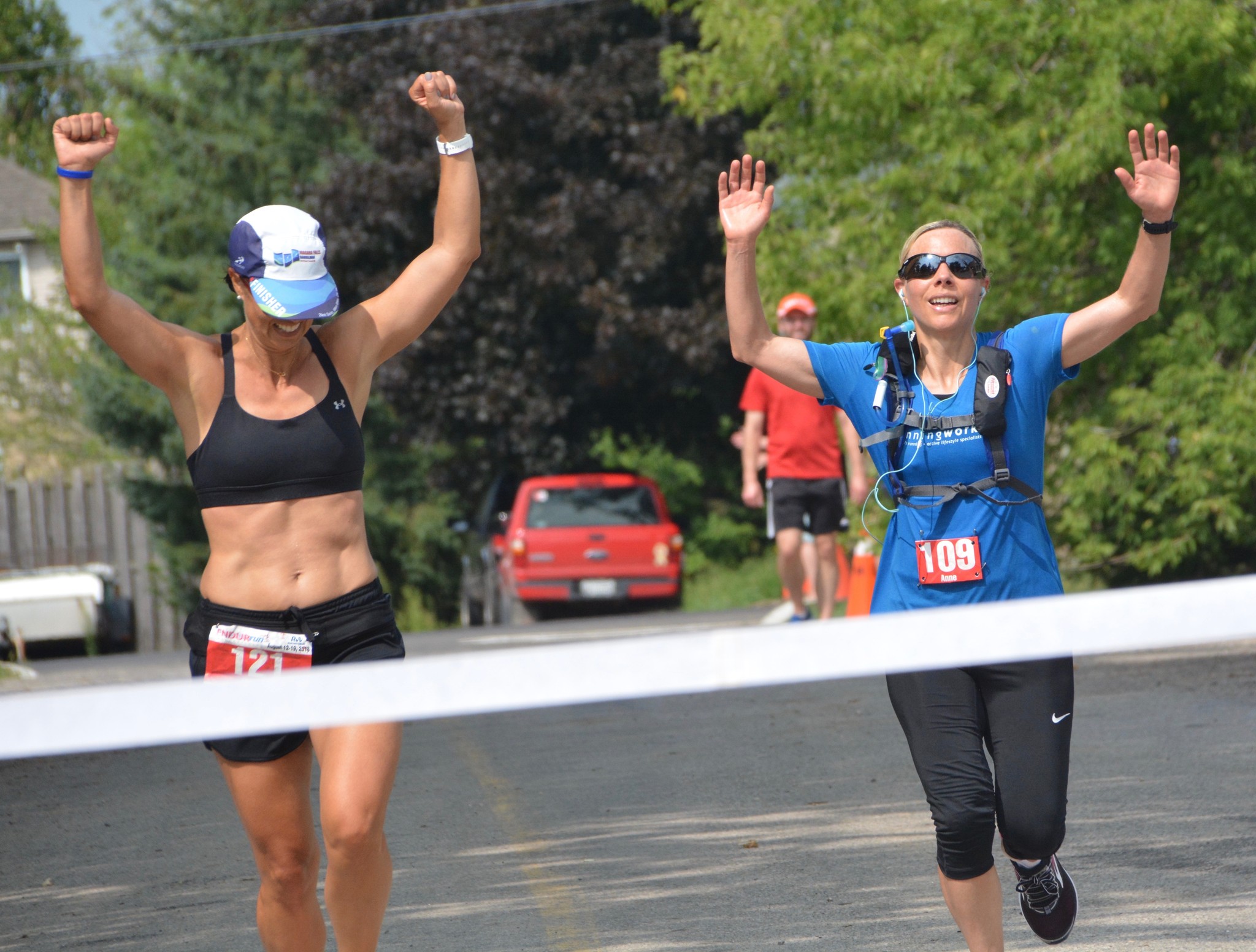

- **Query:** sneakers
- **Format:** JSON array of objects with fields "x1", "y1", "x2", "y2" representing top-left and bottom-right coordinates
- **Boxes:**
[{"x1": 1010, "y1": 856, "x2": 1077, "y2": 945}]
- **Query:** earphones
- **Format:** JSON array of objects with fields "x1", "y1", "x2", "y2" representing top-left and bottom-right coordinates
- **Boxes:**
[
  {"x1": 899, "y1": 288, "x2": 906, "y2": 301},
  {"x1": 980, "y1": 287, "x2": 986, "y2": 299}
]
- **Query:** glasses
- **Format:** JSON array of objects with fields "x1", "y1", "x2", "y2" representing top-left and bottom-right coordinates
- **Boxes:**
[{"x1": 897, "y1": 252, "x2": 989, "y2": 280}]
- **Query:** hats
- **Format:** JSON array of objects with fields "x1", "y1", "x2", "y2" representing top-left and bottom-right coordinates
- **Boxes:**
[
  {"x1": 776, "y1": 292, "x2": 817, "y2": 319},
  {"x1": 227, "y1": 204, "x2": 339, "y2": 319}
]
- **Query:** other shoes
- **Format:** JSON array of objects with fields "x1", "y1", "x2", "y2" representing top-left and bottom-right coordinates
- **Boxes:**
[{"x1": 789, "y1": 607, "x2": 812, "y2": 623}]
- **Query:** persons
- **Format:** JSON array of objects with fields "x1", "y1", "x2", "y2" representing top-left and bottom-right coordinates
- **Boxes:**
[
  {"x1": 51, "y1": 69, "x2": 482, "y2": 951},
  {"x1": 732, "y1": 292, "x2": 870, "y2": 624},
  {"x1": 717, "y1": 122, "x2": 1181, "y2": 952}
]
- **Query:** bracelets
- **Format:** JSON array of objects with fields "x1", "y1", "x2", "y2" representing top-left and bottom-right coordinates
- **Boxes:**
[
  {"x1": 1140, "y1": 212, "x2": 1179, "y2": 235},
  {"x1": 435, "y1": 132, "x2": 474, "y2": 156},
  {"x1": 56, "y1": 166, "x2": 94, "y2": 179}
]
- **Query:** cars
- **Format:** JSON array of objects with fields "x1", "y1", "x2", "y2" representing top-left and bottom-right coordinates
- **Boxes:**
[{"x1": 491, "y1": 472, "x2": 686, "y2": 621}]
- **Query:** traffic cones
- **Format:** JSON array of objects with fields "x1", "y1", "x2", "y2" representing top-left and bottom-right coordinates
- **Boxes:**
[
  {"x1": 781, "y1": 574, "x2": 812, "y2": 602},
  {"x1": 826, "y1": 541, "x2": 850, "y2": 603},
  {"x1": 843, "y1": 530, "x2": 883, "y2": 617}
]
